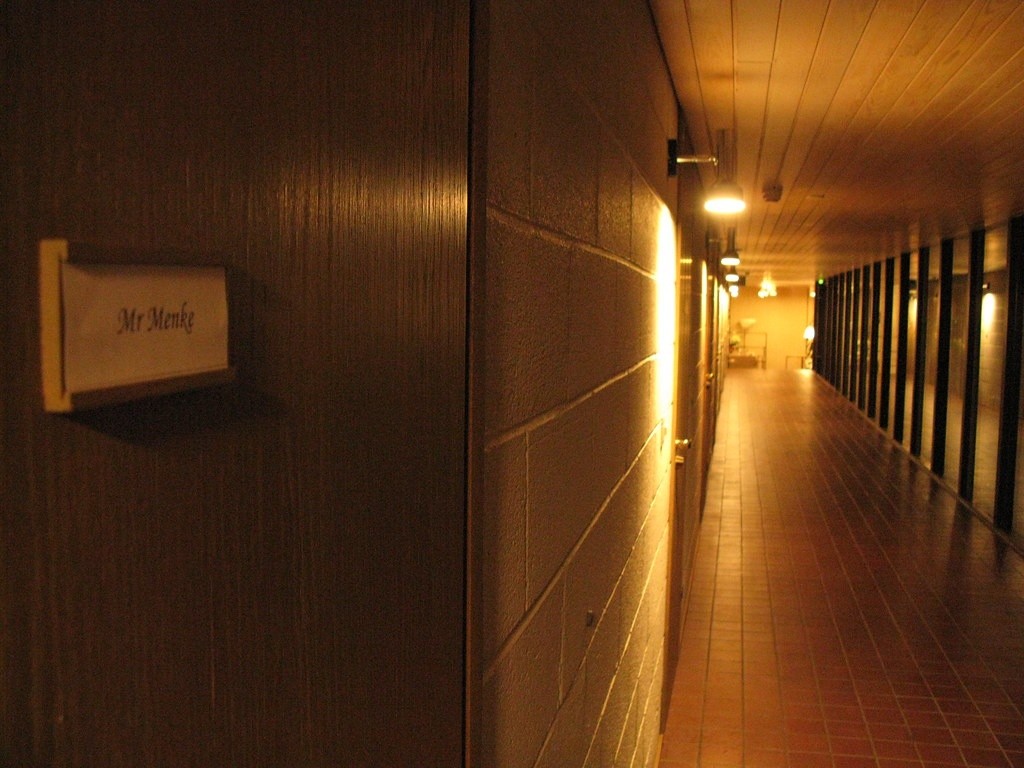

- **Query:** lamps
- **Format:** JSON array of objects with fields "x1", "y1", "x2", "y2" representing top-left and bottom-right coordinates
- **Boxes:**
[
  {"x1": 705, "y1": 225, "x2": 740, "y2": 265},
  {"x1": 667, "y1": 127, "x2": 745, "y2": 215},
  {"x1": 721, "y1": 265, "x2": 740, "y2": 282}
]
